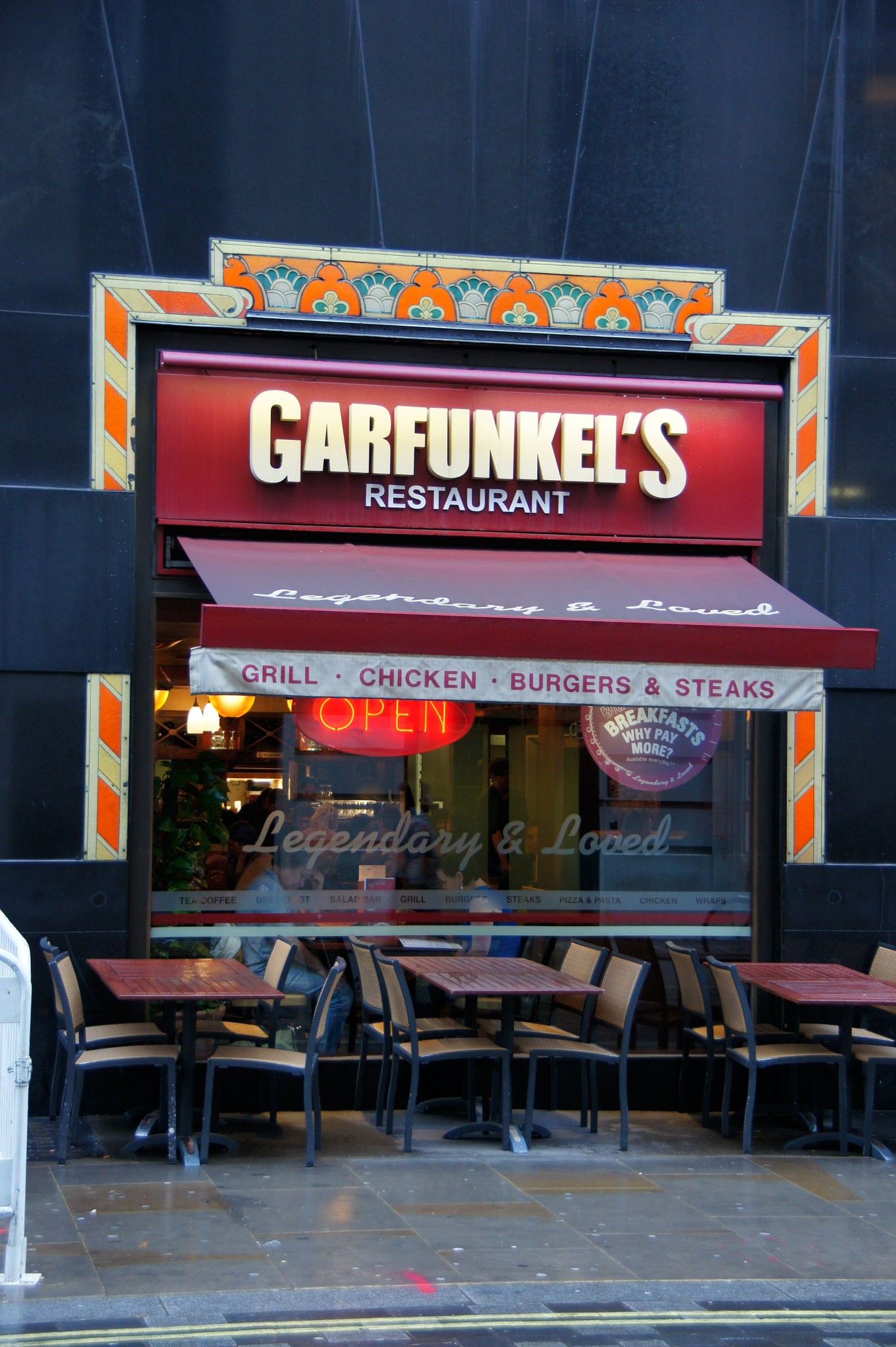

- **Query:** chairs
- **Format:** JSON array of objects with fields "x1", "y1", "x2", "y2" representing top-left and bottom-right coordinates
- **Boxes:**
[
  {"x1": 850, "y1": 1044, "x2": 896, "y2": 1156},
  {"x1": 50, "y1": 951, "x2": 180, "y2": 1165},
  {"x1": 40, "y1": 937, "x2": 171, "y2": 1125},
  {"x1": 518, "y1": 954, "x2": 649, "y2": 1152},
  {"x1": 349, "y1": 934, "x2": 476, "y2": 1125},
  {"x1": 177, "y1": 941, "x2": 298, "y2": 1133},
  {"x1": 478, "y1": 938, "x2": 609, "y2": 1128},
  {"x1": 706, "y1": 952, "x2": 844, "y2": 1152},
  {"x1": 798, "y1": 940, "x2": 896, "y2": 1129},
  {"x1": 376, "y1": 955, "x2": 512, "y2": 1154},
  {"x1": 667, "y1": 938, "x2": 792, "y2": 1127},
  {"x1": 197, "y1": 958, "x2": 345, "y2": 1166}
]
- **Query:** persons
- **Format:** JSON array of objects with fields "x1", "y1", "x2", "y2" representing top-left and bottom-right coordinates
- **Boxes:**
[
  {"x1": 514, "y1": 821, "x2": 545, "y2": 888},
  {"x1": 435, "y1": 849, "x2": 522, "y2": 1000},
  {"x1": 224, "y1": 840, "x2": 356, "y2": 1054},
  {"x1": 202, "y1": 777, "x2": 438, "y2": 899}
]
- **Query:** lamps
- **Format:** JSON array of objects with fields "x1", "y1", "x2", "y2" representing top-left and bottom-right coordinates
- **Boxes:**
[{"x1": 185, "y1": 693, "x2": 255, "y2": 737}]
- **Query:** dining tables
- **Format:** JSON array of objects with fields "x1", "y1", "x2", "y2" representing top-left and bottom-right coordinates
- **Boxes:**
[
  {"x1": 728, "y1": 959, "x2": 896, "y2": 1160},
  {"x1": 382, "y1": 954, "x2": 603, "y2": 1152},
  {"x1": 89, "y1": 956, "x2": 284, "y2": 1168}
]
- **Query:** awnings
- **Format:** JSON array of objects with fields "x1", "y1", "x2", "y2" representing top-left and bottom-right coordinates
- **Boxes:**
[{"x1": 172, "y1": 528, "x2": 881, "y2": 712}]
